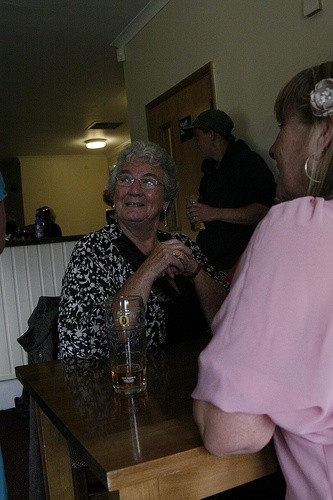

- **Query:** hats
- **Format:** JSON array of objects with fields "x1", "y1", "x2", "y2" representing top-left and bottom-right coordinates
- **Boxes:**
[{"x1": 182, "y1": 109, "x2": 234, "y2": 137}]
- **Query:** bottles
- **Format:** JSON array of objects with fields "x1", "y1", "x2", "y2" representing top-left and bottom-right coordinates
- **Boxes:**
[{"x1": 34, "y1": 211, "x2": 45, "y2": 240}]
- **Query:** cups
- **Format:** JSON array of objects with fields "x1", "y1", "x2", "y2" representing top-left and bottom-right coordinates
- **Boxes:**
[
  {"x1": 105, "y1": 294, "x2": 147, "y2": 395},
  {"x1": 184, "y1": 195, "x2": 206, "y2": 232}
]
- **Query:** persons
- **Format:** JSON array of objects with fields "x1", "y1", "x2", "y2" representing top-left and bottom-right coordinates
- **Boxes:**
[
  {"x1": 0, "y1": 172, "x2": 9, "y2": 255},
  {"x1": 56, "y1": 141, "x2": 231, "y2": 362},
  {"x1": 189, "y1": 62, "x2": 333, "y2": 500},
  {"x1": 183, "y1": 110, "x2": 278, "y2": 275}
]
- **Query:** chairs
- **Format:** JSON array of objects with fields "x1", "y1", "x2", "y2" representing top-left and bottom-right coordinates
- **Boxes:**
[{"x1": 17, "y1": 296, "x2": 111, "y2": 500}]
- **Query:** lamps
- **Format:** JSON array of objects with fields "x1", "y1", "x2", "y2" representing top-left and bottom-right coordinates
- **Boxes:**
[{"x1": 83, "y1": 138, "x2": 107, "y2": 149}]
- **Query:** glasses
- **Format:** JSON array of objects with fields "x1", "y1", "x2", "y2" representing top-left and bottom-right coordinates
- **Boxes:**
[{"x1": 116, "y1": 175, "x2": 165, "y2": 190}]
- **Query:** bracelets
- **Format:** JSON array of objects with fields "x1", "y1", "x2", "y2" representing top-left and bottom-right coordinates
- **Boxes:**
[{"x1": 189, "y1": 263, "x2": 202, "y2": 279}]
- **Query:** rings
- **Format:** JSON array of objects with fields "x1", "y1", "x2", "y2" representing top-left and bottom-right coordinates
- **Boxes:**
[{"x1": 172, "y1": 251, "x2": 181, "y2": 257}]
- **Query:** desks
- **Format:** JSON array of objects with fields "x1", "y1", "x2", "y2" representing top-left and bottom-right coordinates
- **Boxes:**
[{"x1": 14, "y1": 348, "x2": 281, "y2": 500}]
